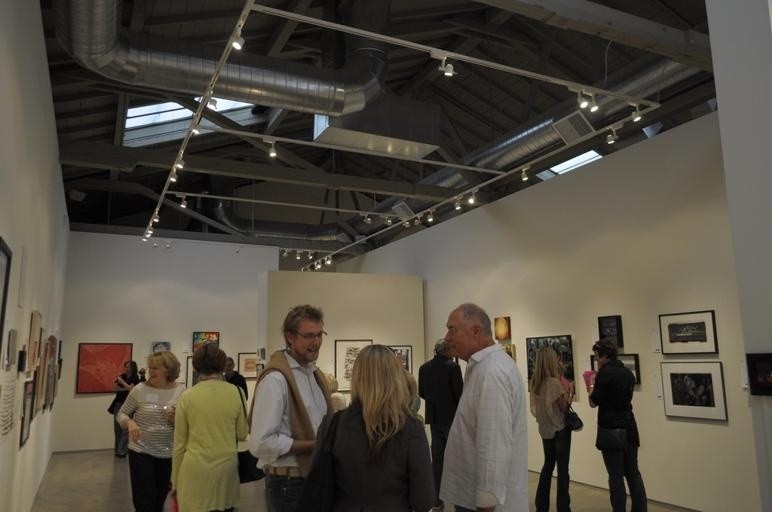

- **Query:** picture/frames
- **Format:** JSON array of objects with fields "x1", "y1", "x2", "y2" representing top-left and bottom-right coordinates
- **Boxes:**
[
  {"x1": 192, "y1": 332, "x2": 219, "y2": 353},
  {"x1": 334, "y1": 339, "x2": 373, "y2": 392},
  {"x1": 658, "y1": 310, "x2": 719, "y2": 355},
  {"x1": 590, "y1": 354, "x2": 641, "y2": 384},
  {"x1": 660, "y1": 360, "x2": 729, "y2": 421},
  {"x1": 19, "y1": 380, "x2": 35, "y2": 446},
  {"x1": 238, "y1": 353, "x2": 257, "y2": 378},
  {"x1": 76, "y1": 343, "x2": 133, "y2": 394},
  {"x1": 387, "y1": 345, "x2": 413, "y2": 375},
  {"x1": 186, "y1": 356, "x2": 200, "y2": 389},
  {"x1": 0, "y1": 235, "x2": 14, "y2": 356}
]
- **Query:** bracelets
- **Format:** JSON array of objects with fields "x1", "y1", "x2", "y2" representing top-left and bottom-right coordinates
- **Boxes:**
[
  {"x1": 568, "y1": 392, "x2": 573, "y2": 398},
  {"x1": 588, "y1": 384, "x2": 594, "y2": 388},
  {"x1": 588, "y1": 389, "x2": 593, "y2": 392}
]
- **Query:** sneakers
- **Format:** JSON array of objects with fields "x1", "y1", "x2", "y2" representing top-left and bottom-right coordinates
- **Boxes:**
[{"x1": 116, "y1": 453, "x2": 126, "y2": 457}]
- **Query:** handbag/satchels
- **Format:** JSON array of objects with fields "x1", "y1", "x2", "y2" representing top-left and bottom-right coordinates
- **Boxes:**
[
  {"x1": 565, "y1": 410, "x2": 583, "y2": 432},
  {"x1": 595, "y1": 425, "x2": 627, "y2": 451}
]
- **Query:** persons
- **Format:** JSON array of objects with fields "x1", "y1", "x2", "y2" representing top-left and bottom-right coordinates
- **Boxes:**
[
  {"x1": 222, "y1": 356, "x2": 249, "y2": 450},
  {"x1": 108, "y1": 361, "x2": 139, "y2": 457},
  {"x1": 116, "y1": 350, "x2": 185, "y2": 512},
  {"x1": 249, "y1": 303, "x2": 332, "y2": 510},
  {"x1": 529, "y1": 345, "x2": 576, "y2": 511},
  {"x1": 587, "y1": 338, "x2": 648, "y2": 511},
  {"x1": 437, "y1": 302, "x2": 530, "y2": 511},
  {"x1": 162, "y1": 342, "x2": 250, "y2": 512},
  {"x1": 418, "y1": 338, "x2": 464, "y2": 510},
  {"x1": 303, "y1": 345, "x2": 439, "y2": 511}
]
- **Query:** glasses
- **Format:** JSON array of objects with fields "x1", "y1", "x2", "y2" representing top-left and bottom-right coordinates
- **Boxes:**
[{"x1": 295, "y1": 332, "x2": 325, "y2": 339}]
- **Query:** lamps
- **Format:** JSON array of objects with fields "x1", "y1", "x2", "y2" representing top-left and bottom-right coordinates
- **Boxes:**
[
  {"x1": 180, "y1": 201, "x2": 187, "y2": 208},
  {"x1": 268, "y1": 148, "x2": 276, "y2": 157},
  {"x1": 232, "y1": 37, "x2": 245, "y2": 50},
  {"x1": 444, "y1": 63, "x2": 454, "y2": 76},
  {"x1": 169, "y1": 158, "x2": 184, "y2": 182},
  {"x1": 192, "y1": 125, "x2": 201, "y2": 136},
  {"x1": 364, "y1": 214, "x2": 434, "y2": 228},
  {"x1": 454, "y1": 195, "x2": 477, "y2": 211},
  {"x1": 142, "y1": 214, "x2": 160, "y2": 242},
  {"x1": 282, "y1": 249, "x2": 333, "y2": 271},
  {"x1": 521, "y1": 170, "x2": 528, "y2": 181},
  {"x1": 579, "y1": 90, "x2": 643, "y2": 145}
]
